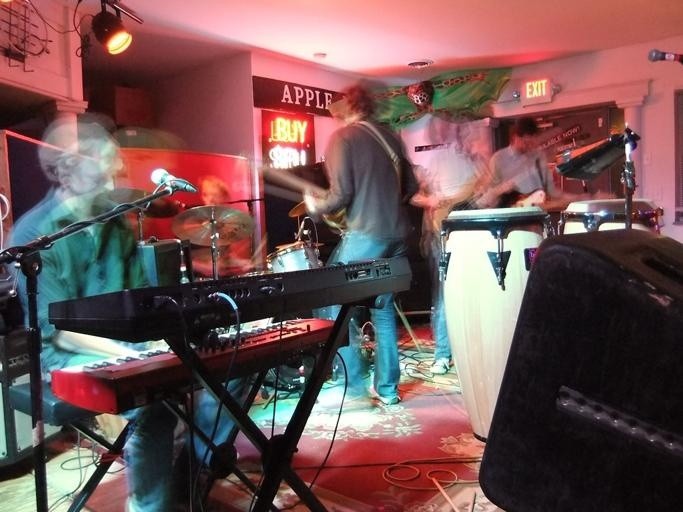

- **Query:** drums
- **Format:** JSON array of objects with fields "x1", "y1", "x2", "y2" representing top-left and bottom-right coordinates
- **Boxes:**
[
  {"x1": 267, "y1": 241, "x2": 325, "y2": 274},
  {"x1": 441, "y1": 206, "x2": 557, "y2": 444},
  {"x1": 557, "y1": 198, "x2": 664, "y2": 236}
]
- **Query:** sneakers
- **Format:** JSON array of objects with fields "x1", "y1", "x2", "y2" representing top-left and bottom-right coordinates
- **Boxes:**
[
  {"x1": 317, "y1": 386, "x2": 401, "y2": 411},
  {"x1": 430, "y1": 360, "x2": 450, "y2": 374}
]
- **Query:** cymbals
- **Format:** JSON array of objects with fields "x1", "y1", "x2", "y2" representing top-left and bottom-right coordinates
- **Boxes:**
[
  {"x1": 92, "y1": 189, "x2": 180, "y2": 218},
  {"x1": 172, "y1": 204, "x2": 257, "y2": 247}
]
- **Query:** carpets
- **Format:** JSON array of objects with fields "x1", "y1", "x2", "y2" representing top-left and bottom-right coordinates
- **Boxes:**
[{"x1": 85, "y1": 322, "x2": 505, "y2": 512}]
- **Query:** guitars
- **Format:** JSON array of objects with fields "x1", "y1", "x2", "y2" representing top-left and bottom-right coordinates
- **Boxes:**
[
  {"x1": 240, "y1": 149, "x2": 353, "y2": 234},
  {"x1": 501, "y1": 189, "x2": 584, "y2": 210}
]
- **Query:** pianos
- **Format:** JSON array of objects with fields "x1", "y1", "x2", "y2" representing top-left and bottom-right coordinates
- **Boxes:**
[
  {"x1": 51, "y1": 319, "x2": 349, "y2": 414},
  {"x1": 48, "y1": 252, "x2": 412, "y2": 343}
]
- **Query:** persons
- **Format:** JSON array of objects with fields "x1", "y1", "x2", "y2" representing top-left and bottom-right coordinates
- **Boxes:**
[
  {"x1": 410, "y1": 115, "x2": 616, "y2": 375},
  {"x1": 5, "y1": 121, "x2": 252, "y2": 512},
  {"x1": 303, "y1": 79, "x2": 420, "y2": 405}
]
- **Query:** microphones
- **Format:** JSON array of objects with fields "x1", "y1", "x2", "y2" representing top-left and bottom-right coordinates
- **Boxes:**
[
  {"x1": 151, "y1": 168, "x2": 196, "y2": 193},
  {"x1": 295, "y1": 220, "x2": 305, "y2": 241},
  {"x1": 648, "y1": 48, "x2": 683, "y2": 65}
]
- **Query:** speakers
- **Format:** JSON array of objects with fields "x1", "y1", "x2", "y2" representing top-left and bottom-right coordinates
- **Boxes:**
[
  {"x1": 478, "y1": 229, "x2": 683, "y2": 510},
  {"x1": 137, "y1": 237, "x2": 192, "y2": 287}
]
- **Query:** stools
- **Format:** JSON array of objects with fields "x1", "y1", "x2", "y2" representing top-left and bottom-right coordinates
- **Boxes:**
[{"x1": 8, "y1": 382, "x2": 138, "y2": 510}]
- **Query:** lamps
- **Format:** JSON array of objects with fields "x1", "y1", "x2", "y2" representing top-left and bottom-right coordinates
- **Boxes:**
[{"x1": 91, "y1": 0, "x2": 133, "y2": 55}]
